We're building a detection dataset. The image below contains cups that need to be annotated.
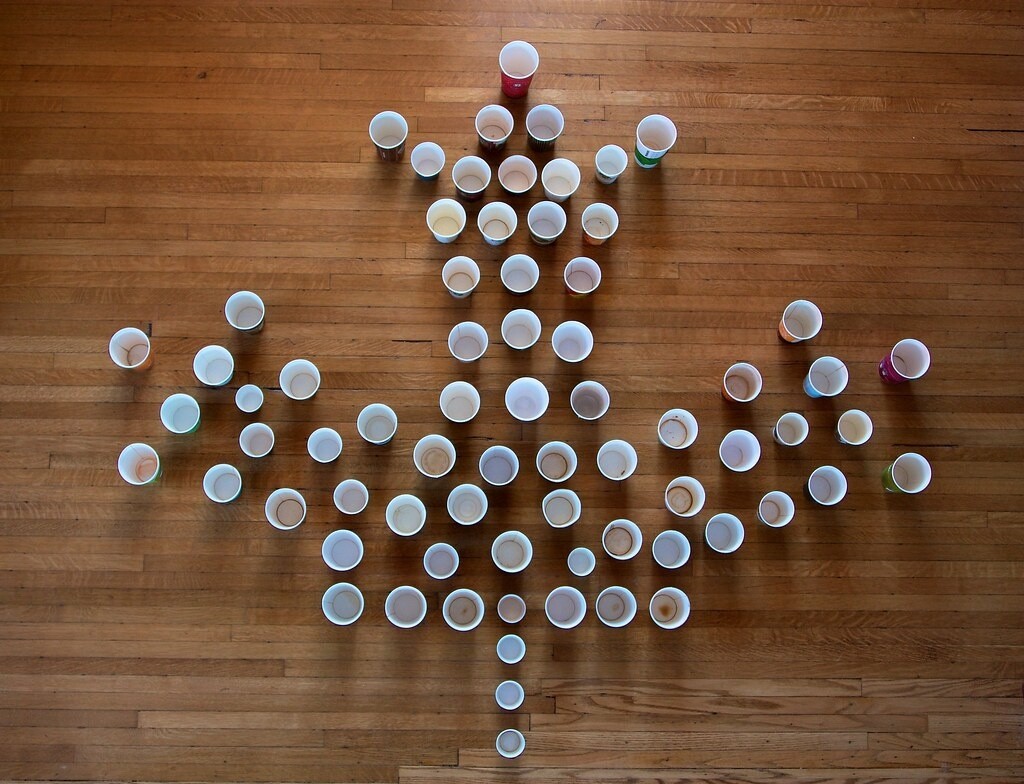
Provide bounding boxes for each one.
[109,41,932,759]
